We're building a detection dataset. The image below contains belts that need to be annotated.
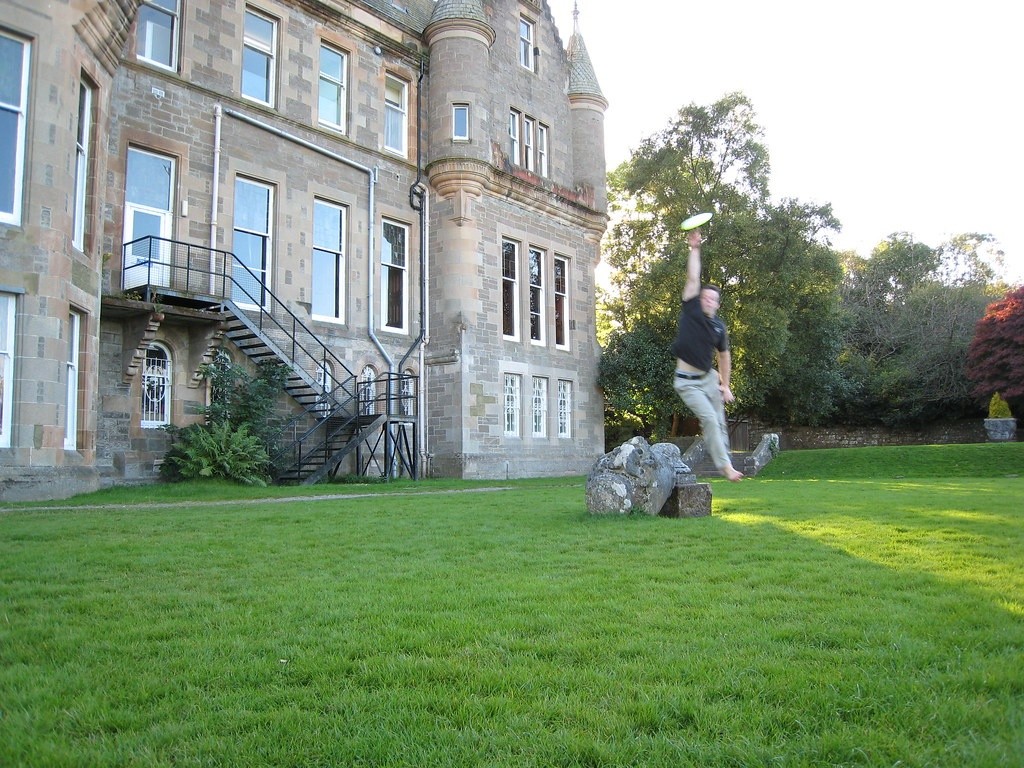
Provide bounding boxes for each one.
[674,372,708,380]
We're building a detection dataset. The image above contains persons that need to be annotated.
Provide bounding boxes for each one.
[672,228,744,483]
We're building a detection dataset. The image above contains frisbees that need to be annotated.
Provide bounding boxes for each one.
[680,213,713,231]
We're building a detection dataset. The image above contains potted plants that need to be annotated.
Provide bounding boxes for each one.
[984,392,1018,442]
